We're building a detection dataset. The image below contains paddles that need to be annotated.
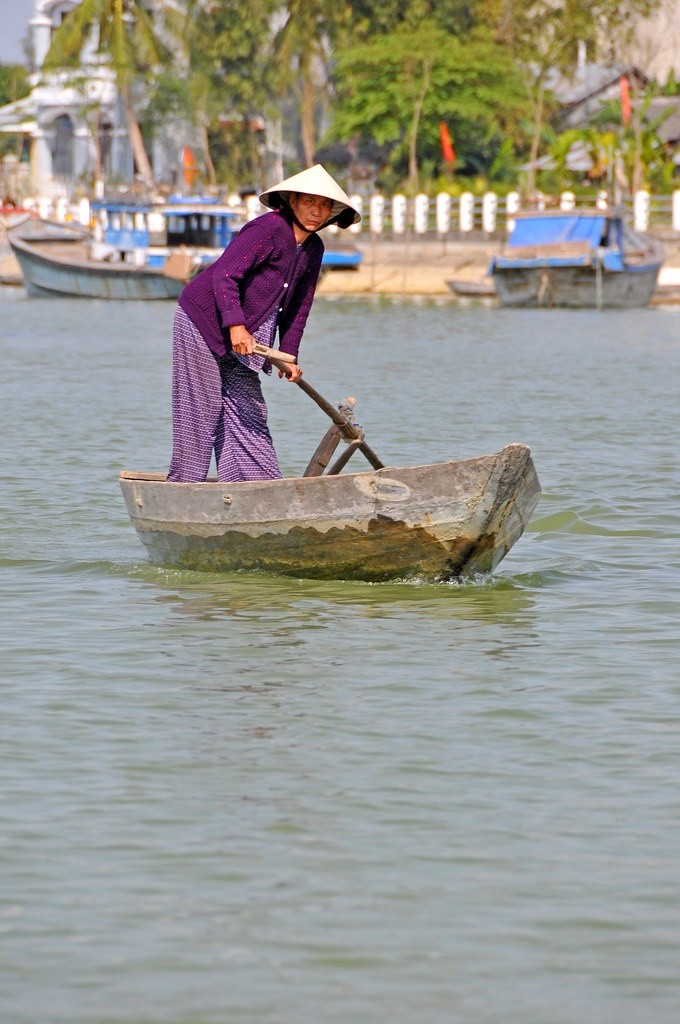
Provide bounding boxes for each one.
[251,342,384,470]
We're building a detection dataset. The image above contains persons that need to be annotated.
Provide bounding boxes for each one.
[166,163,361,482]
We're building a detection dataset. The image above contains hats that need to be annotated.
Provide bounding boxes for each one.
[257,164,362,233]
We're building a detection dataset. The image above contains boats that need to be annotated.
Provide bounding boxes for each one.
[485,210,665,306]
[118,443,542,587]
[6,204,363,299]
[446,280,494,296]
